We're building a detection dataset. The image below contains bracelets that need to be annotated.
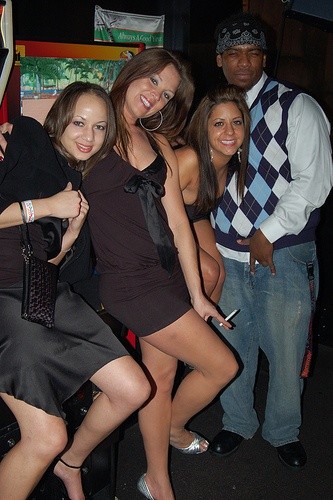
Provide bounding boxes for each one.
[18,201,26,224]
[24,200,34,223]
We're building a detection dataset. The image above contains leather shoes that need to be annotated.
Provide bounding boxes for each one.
[277,441,307,471]
[210,430,244,456]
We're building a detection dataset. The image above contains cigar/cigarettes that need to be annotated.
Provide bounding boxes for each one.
[218,309,239,326]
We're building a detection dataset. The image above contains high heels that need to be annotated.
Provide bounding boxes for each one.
[170,428,210,455]
[46,458,86,500]
[137,472,175,500]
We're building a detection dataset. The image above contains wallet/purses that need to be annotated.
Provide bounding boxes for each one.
[18,201,59,328]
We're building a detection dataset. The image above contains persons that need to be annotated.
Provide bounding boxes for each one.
[0,48,240,500]
[210,19,333,471]
[172,83,251,301]
[0,81,151,500]
